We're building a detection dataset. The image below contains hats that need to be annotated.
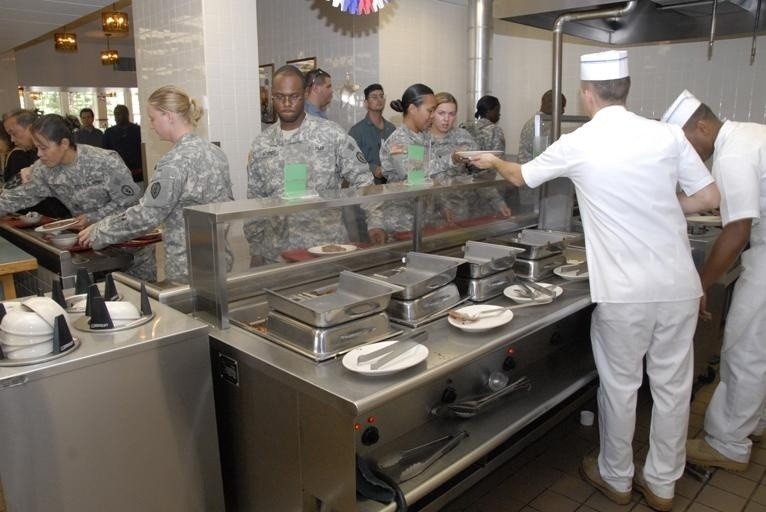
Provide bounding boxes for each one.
[660,88,702,129]
[580,50,629,81]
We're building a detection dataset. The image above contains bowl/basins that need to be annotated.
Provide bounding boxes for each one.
[50,233,79,252]
[1,296,141,364]
[19,214,43,225]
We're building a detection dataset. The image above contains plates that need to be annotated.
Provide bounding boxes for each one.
[687,215,724,227]
[142,229,162,242]
[339,264,588,378]
[307,244,357,255]
[33,217,80,234]
[454,149,504,161]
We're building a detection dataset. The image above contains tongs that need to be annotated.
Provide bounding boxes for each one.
[560,260,587,278]
[513,272,555,302]
[685,464,713,484]
[356,328,431,371]
[375,430,469,487]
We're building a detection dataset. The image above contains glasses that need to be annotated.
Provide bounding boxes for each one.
[310,68,324,87]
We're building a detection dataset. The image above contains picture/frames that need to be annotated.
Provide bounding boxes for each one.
[259,64,276,124]
[287,56,316,79]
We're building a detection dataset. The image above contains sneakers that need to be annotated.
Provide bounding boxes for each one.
[579,455,632,505]
[686,437,749,472]
[633,459,674,511]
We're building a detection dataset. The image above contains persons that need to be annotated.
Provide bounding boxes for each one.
[243,65,511,265]
[79,85,234,278]
[466,50,719,511]
[662,90,766,472]
[1,104,144,231]
[518,90,567,211]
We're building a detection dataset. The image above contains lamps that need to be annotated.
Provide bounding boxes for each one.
[102,3,129,33]
[101,34,118,65]
[55,26,77,53]
